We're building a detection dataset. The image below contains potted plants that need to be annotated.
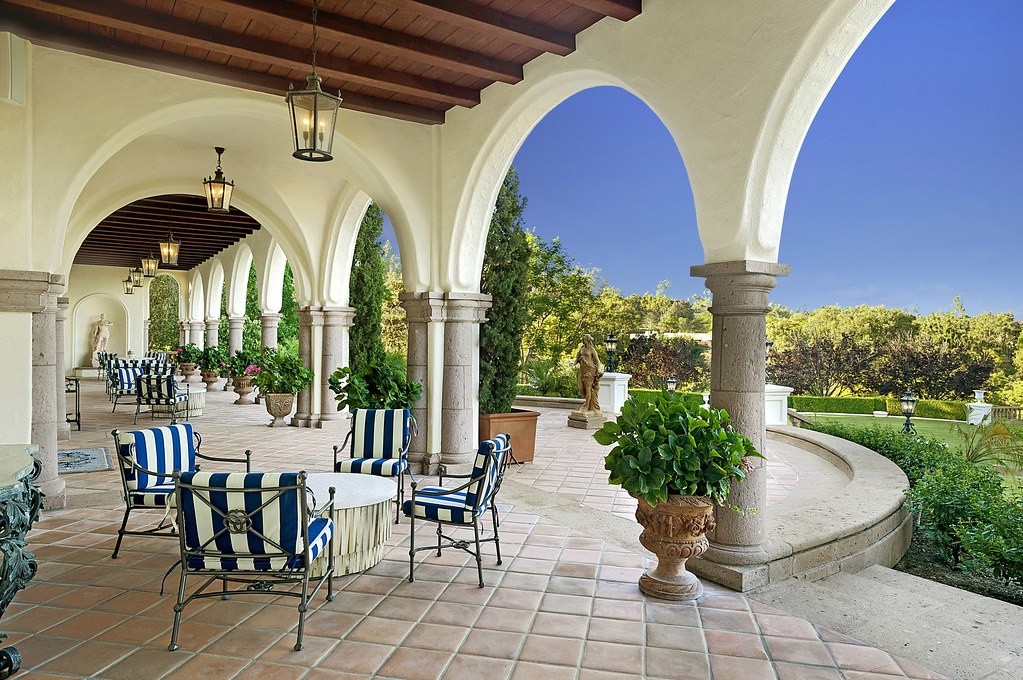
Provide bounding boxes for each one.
[198,346,226,391]
[229,350,259,405]
[173,343,203,383]
[478,167,541,467]
[591,391,767,601]
[249,355,314,428]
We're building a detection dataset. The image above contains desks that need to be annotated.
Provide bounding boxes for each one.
[303,473,399,579]
[151,383,206,418]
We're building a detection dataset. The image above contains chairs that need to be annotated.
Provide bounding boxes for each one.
[97,350,512,652]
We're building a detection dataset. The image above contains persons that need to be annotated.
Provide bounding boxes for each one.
[573,334,601,412]
[94,314,114,352]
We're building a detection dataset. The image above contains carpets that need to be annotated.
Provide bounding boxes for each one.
[58,447,116,474]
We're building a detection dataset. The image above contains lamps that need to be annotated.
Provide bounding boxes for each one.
[284,0,343,161]
[131,248,144,287]
[899,387,919,435]
[158,204,181,267]
[122,265,134,294]
[140,233,160,277]
[202,147,234,214]
